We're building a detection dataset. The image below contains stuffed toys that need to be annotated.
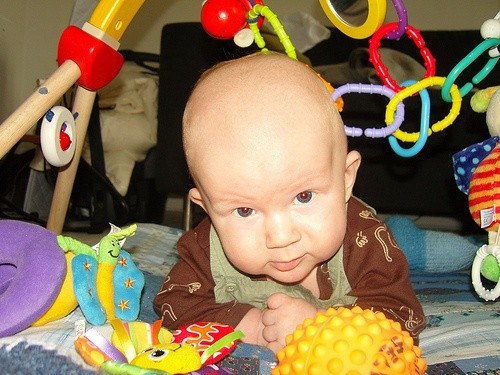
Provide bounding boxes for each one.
[454,85,500,282]
[0,219,247,375]
[56,218,145,326]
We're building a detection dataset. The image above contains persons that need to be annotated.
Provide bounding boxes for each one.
[150,50,427,345]
